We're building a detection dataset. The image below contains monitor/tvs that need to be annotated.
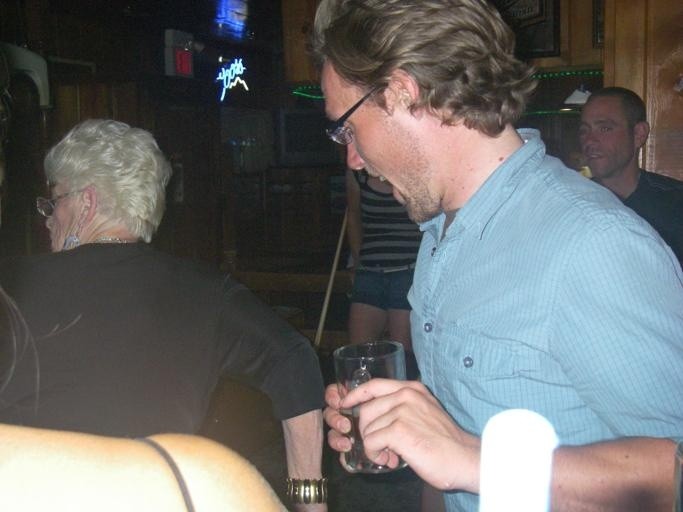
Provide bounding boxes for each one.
[212,0,249,42]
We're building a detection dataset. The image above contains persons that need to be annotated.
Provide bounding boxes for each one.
[577,86,682,270]
[0,118,329,512]
[309,0,683,512]
[0,423,289,511]
[343,164,423,378]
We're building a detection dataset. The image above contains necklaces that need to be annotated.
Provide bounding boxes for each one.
[92,236,127,243]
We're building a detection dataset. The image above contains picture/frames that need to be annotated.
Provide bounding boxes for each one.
[494,0,561,60]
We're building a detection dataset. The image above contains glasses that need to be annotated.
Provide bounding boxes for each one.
[36,193,69,217]
[324,83,387,146]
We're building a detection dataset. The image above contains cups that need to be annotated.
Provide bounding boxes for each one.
[333,340,409,474]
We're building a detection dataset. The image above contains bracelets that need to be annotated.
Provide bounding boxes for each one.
[285,478,329,504]
